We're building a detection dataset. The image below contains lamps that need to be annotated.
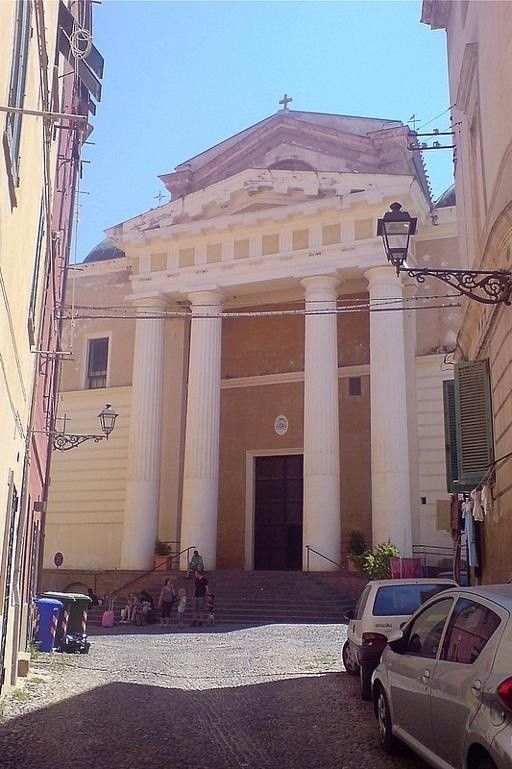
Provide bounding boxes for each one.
[375,202,512,307]
[52,403,119,453]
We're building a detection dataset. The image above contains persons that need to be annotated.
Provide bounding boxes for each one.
[190,569,209,627]
[205,592,216,627]
[88,589,96,605]
[119,592,138,624]
[127,591,153,625]
[186,551,203,579]
[175,589,187,629]
[157,578,176,628]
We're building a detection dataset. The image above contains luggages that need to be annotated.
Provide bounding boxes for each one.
[101,599,115,627]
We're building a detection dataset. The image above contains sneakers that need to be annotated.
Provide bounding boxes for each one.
[118,621,127,625]
[160,622,216,629]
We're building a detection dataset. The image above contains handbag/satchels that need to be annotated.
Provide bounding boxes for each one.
[172,594,177,602]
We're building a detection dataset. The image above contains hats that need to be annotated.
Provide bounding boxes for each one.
[206,592,213,597]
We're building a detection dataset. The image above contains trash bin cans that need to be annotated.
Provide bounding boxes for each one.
[41,590,76,648]
[63,592,92,641]
[32,597,63,653]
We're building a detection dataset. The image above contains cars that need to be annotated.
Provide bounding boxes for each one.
[342,578,460,699]
[371,584,512,768]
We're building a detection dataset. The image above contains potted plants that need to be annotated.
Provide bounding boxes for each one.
[345,530,366,573]
[154,542,173,571]
